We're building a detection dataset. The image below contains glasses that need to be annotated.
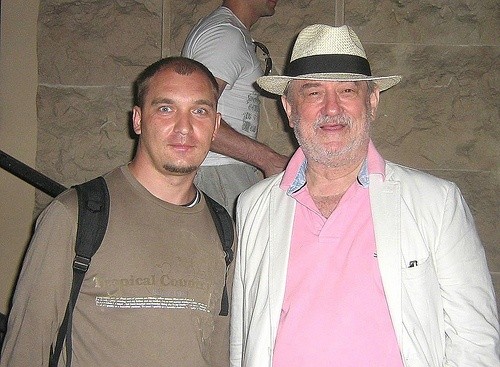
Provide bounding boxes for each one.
[253,40,273,76]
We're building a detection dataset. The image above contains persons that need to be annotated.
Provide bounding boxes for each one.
[229,23,500,367]
[0,56,239,367]
[179,0,291,222]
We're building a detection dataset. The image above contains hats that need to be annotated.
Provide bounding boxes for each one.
[256,24,403,95]
